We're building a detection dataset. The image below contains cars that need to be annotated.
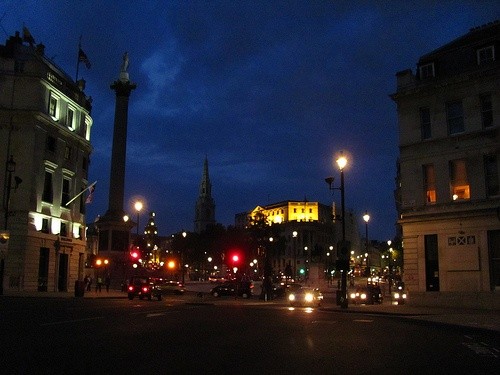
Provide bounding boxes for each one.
[289,287,324,308]
[394,281,406,305]
[127,276,186,301]
[351,284,384,305]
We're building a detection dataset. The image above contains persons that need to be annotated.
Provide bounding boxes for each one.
[105,276,112,292]
[84,274,93,291]
[95,275,103,293]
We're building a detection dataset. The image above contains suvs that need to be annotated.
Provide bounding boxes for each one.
[209,279,255,299]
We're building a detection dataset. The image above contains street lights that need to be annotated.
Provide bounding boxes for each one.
[324,150,349,309]
[133,199,143,276]
[122,215,129,273]
[361,209,371,304]
[181,231,187,285]
[387,239,393,295]
[291,229,298,291]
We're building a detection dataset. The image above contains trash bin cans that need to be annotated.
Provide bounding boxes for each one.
[336,290,342,305]
[75,280,84,297]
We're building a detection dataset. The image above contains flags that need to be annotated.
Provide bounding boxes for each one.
[79,49,92,70]
[86,183,95,204]
[22,27,35,45]
